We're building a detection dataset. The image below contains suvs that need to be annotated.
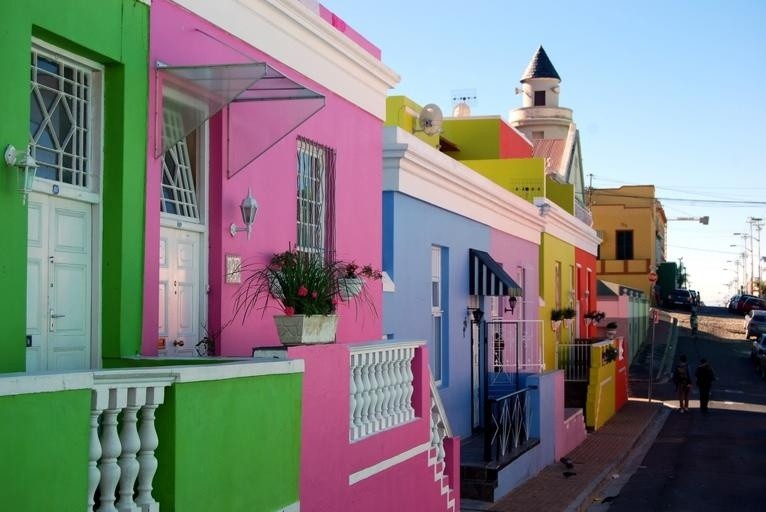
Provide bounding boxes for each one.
[744,311,766,340]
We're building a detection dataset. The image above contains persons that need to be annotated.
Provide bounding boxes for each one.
[671,355,692,414]
[695,358,716,414]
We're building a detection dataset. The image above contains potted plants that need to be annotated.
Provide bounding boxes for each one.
[592,312,605,326]
[563,308,577,328]
[217,242,379,344]
[550,308,564,330]
[584,311,596,326]
[337,261,383,301]
[606,322,617,338]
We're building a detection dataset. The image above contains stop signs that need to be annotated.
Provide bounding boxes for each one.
[648,273,657,282]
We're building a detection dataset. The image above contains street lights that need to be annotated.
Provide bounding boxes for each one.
[678,257,687,289]
[723,216,762,299]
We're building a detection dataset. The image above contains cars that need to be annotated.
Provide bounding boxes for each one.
[668,290,698,309]
[729,293,765,313]
[752,332,766,364]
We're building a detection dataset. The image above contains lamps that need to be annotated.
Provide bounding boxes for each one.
[471,308,484,328]
[505,296,517,315]
[5,144,39,204]
[229,187,258,240]
